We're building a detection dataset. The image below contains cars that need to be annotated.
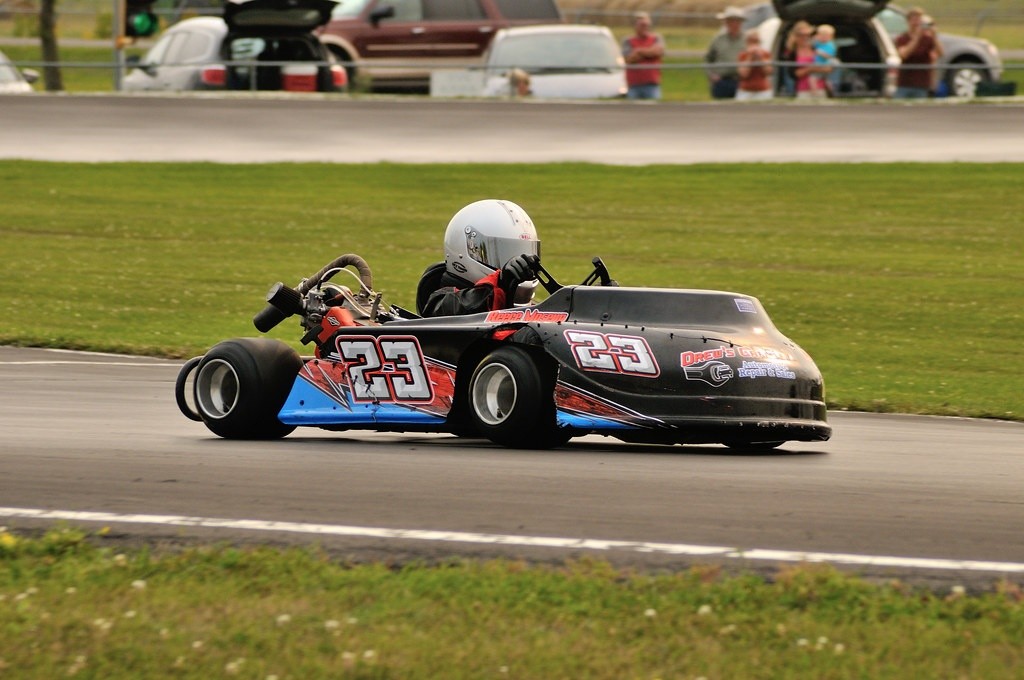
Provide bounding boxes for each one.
[742,1,1005,98]
[478,25,630,99]
[0,51,40,95]
[117,19,346,94]
[769,0,901,99]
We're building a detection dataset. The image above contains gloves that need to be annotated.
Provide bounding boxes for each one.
[497,253,540,292]
[595,279,619,286]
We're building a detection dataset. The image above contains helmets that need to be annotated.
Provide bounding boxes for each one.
[444,199,540,289]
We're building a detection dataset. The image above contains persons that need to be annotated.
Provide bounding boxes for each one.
[621,11,666,100]
[416,199,619,317]
[702,0,944,101]
[510,70,533,96]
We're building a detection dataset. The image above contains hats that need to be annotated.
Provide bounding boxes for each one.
[715,7,748,20]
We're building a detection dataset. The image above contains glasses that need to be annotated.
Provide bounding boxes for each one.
[796,32,815,38]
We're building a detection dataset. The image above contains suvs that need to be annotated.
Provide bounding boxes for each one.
[313,0,570,94]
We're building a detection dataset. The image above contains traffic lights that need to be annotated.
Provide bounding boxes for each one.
[116,0,157,46]
